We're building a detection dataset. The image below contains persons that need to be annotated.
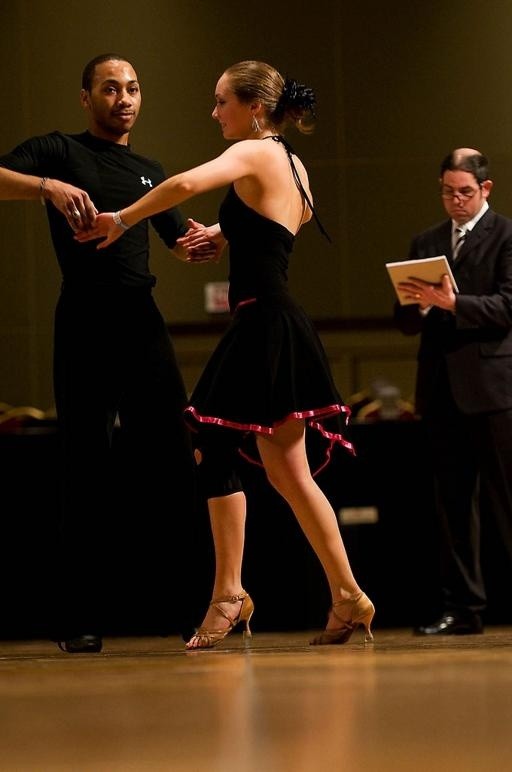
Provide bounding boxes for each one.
[0,54,217,652]
[395,148,512,636]
[74,60,377,650]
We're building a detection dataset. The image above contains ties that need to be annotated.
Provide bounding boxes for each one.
[448,229,465,259]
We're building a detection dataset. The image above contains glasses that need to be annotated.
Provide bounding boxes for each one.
[439,179,483,202]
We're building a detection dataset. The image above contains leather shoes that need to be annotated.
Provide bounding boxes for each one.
[61,631,104,653]
[411,602,485,638]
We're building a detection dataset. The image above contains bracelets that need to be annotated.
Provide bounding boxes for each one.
[112,211,130,230]
[40,177,47,201]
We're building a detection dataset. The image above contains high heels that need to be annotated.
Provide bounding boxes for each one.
[307,588,377,647]
[181,586,256,650]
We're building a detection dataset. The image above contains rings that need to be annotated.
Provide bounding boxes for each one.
[416,293,420,298]
[72,210,80,218]
